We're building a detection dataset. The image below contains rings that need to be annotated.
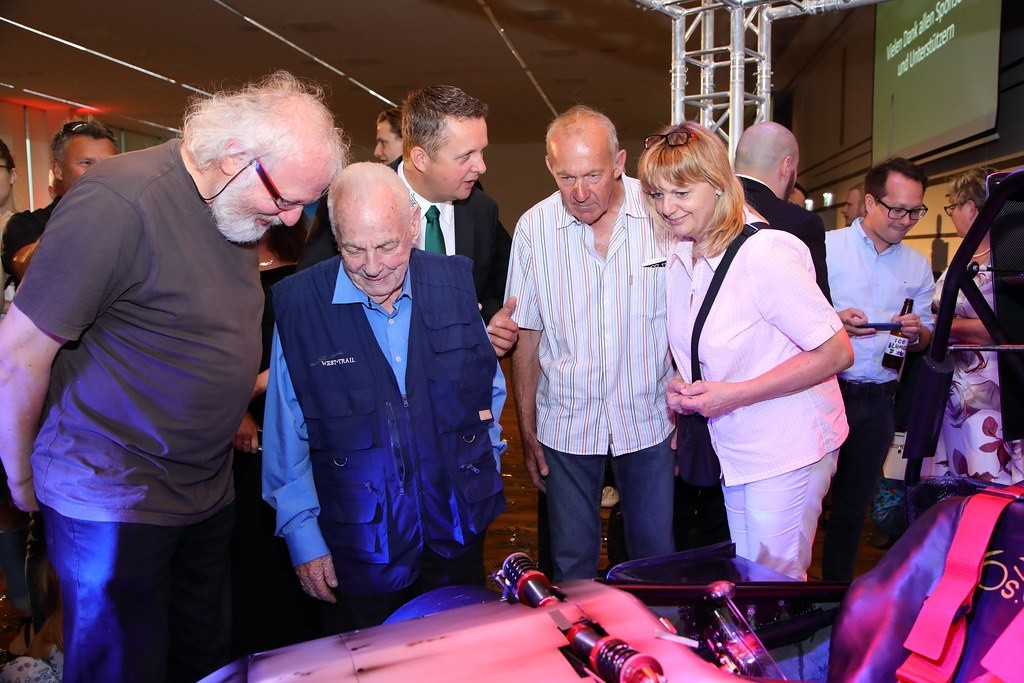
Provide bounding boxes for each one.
[242,441,250,447]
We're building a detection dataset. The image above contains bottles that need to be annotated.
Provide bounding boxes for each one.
[2,281,15,314]
[882,298,915,370]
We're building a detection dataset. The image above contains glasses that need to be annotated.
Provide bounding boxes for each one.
[253,160,328,211]
[871,192,928,220]
[945,202,963,217]
[53,121,113,159]
[643,131,700,146]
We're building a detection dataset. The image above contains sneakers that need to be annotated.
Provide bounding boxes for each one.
[600,486,619,507]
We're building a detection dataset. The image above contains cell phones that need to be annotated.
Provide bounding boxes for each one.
[855,322,902,332]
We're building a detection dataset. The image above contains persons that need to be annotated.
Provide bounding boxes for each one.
[639,111,855,580]
[825,155,935,580]
[24,548,66,682]
[232,209,332,657]
[1,68,368,682]
[502,98,679,582]
[387,83,517,589]
[1,114,122,635]
[263,162,507,638]
[932,165,1024,485]
[0,141,36,656]
[787,188,807,210]
[304,192,340,260]
[373,108,404,174]
[674,120,834,552]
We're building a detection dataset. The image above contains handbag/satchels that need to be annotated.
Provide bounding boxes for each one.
[675,409,721,486]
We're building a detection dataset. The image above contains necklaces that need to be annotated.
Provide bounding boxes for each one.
[973,247,990,257]
[258,254,279,267]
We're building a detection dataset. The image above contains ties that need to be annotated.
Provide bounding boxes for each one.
[423,206,446,256]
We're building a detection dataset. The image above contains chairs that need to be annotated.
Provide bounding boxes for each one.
[827,493,1024,683]
